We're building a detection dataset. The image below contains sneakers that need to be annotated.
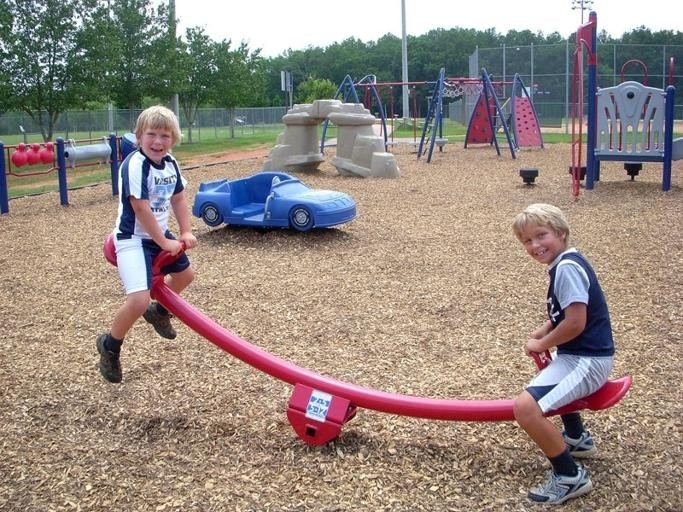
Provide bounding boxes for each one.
[142,300,177,341]
[94,333,125,383]
[557,428,600,459]
[525,464,595,506]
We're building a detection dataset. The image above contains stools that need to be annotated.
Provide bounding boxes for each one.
[104,233,187,274]
[531,350,631,414]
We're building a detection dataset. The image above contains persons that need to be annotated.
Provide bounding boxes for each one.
[93,103,199,384]
[510,200,617,508]
